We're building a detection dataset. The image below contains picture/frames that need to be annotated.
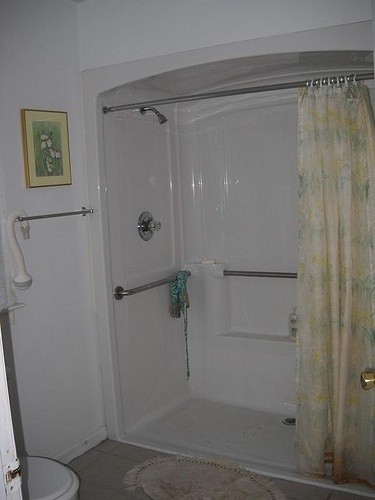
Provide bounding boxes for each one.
[21,109,72,187]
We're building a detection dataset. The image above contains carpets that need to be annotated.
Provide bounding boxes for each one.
[123,454,287,500]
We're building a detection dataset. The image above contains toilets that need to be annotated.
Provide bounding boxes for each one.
[16,455,80,500]
